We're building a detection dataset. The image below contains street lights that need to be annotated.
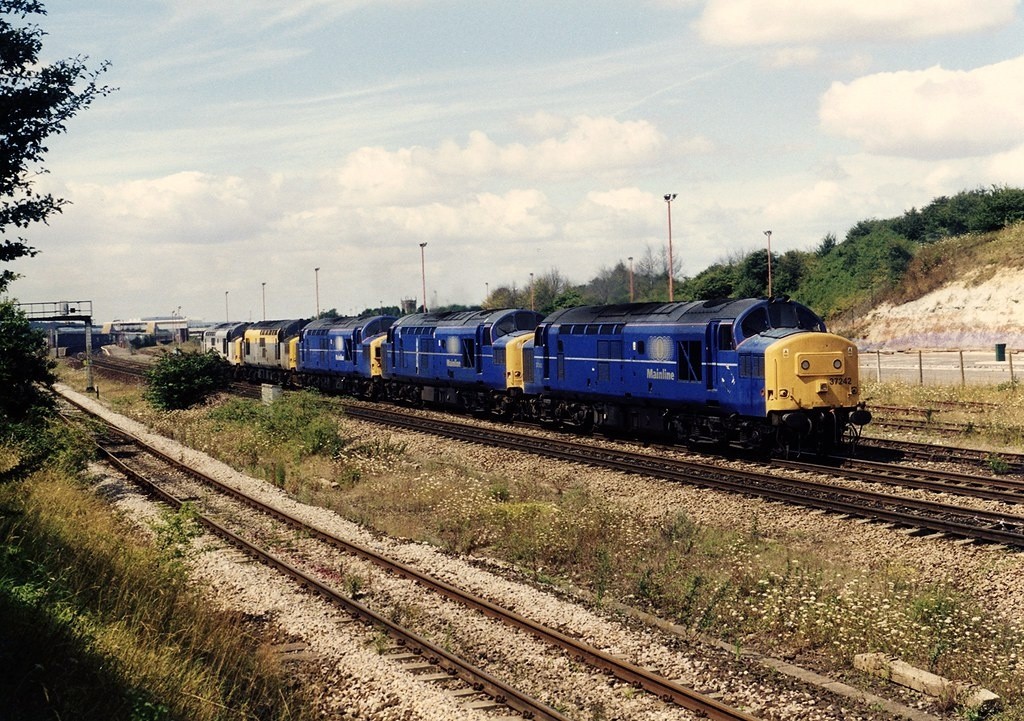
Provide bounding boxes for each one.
[663,192,679,303]
[262,282,266,321]
[627,256,634,304]
[171,306,183,322]
[419,242,427,314]
[530,272,534,311]
[315,267,320,321]
[762,228,773,296]
[225,291,228,323]
[484,282,489,299]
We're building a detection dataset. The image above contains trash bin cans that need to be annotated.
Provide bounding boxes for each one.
[995,343,1006,361]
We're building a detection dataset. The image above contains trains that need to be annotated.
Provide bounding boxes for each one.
[202,296,874,458]
